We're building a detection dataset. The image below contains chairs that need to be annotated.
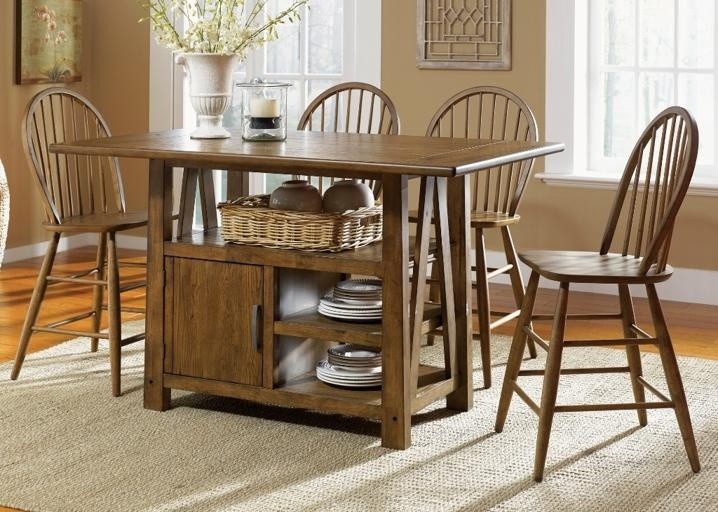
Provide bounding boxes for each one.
[495,106,702,482]
[10,86,180,396]
[408,86,538,387]
[291,81,401,200]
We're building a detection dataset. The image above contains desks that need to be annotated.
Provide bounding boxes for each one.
[47,127,565,450]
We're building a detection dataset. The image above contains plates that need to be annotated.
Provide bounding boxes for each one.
[315,345,383,389]
[318,278,383,322]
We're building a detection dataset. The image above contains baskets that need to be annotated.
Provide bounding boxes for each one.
[216,192,385,254]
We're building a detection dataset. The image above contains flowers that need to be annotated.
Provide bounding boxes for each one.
[131,1,314,63]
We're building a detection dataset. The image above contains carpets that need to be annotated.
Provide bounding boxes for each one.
[0,318,716,511]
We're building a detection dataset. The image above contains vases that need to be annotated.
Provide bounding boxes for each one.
[176,54,243,138]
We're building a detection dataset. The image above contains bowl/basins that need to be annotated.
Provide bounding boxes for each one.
[269,180,374,212]
